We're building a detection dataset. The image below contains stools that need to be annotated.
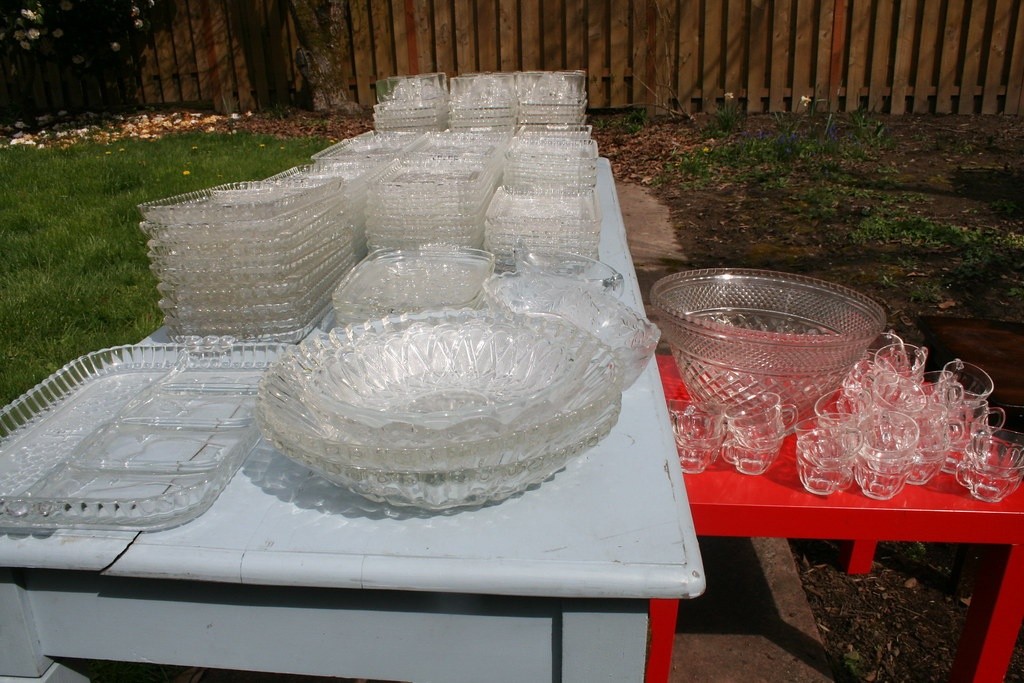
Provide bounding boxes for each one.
[913,313,1024,598]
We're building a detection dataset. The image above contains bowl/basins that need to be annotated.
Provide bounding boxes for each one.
[649,266,887,425]
[256,311,626,512]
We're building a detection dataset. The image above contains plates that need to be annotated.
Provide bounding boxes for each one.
[141,65,661,391]
[1,337,298,535]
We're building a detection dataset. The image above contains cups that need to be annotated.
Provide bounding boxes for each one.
[683,330,1024,503]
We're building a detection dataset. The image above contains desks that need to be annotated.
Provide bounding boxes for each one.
[0,155,707,683]
[643,356,1024,683]
